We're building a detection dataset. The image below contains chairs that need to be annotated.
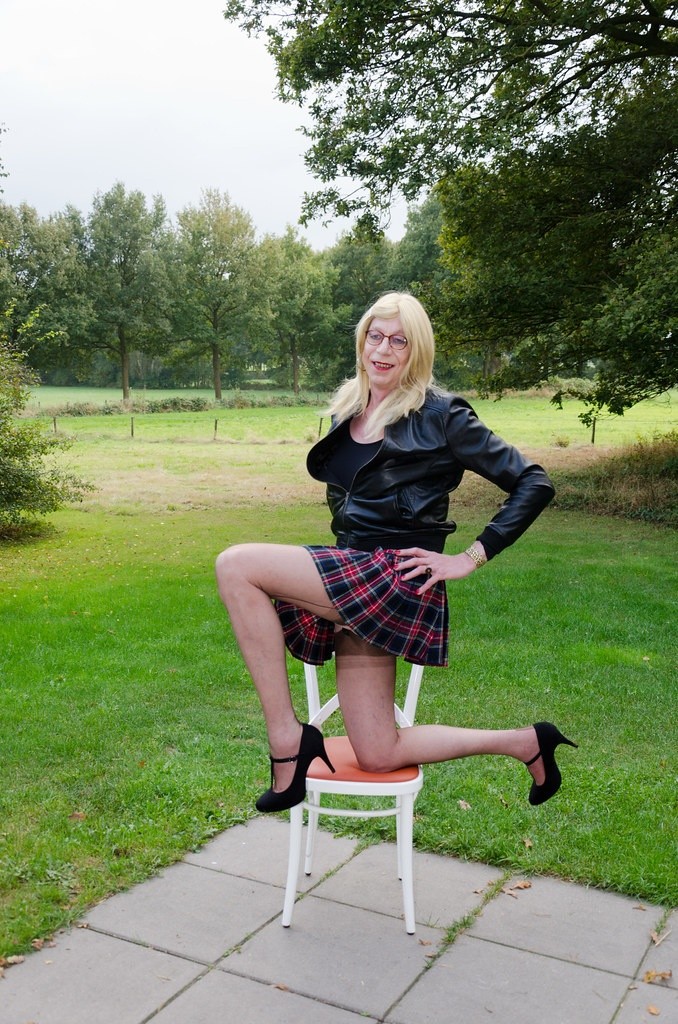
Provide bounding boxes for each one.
[281,653,424,937]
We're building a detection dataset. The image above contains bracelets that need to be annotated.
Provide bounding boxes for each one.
[465,547,485,568]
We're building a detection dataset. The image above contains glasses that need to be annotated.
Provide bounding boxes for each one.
[361,327,412,352]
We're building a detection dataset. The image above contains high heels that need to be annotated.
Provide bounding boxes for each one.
[524,722,579,805]
[255,723,336,814]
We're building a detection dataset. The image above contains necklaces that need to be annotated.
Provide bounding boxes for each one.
[365,410,370,420]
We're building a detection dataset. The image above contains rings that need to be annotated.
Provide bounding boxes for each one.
[425,565,432,574]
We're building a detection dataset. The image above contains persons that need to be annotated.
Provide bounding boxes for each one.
[216,294,579,812]
